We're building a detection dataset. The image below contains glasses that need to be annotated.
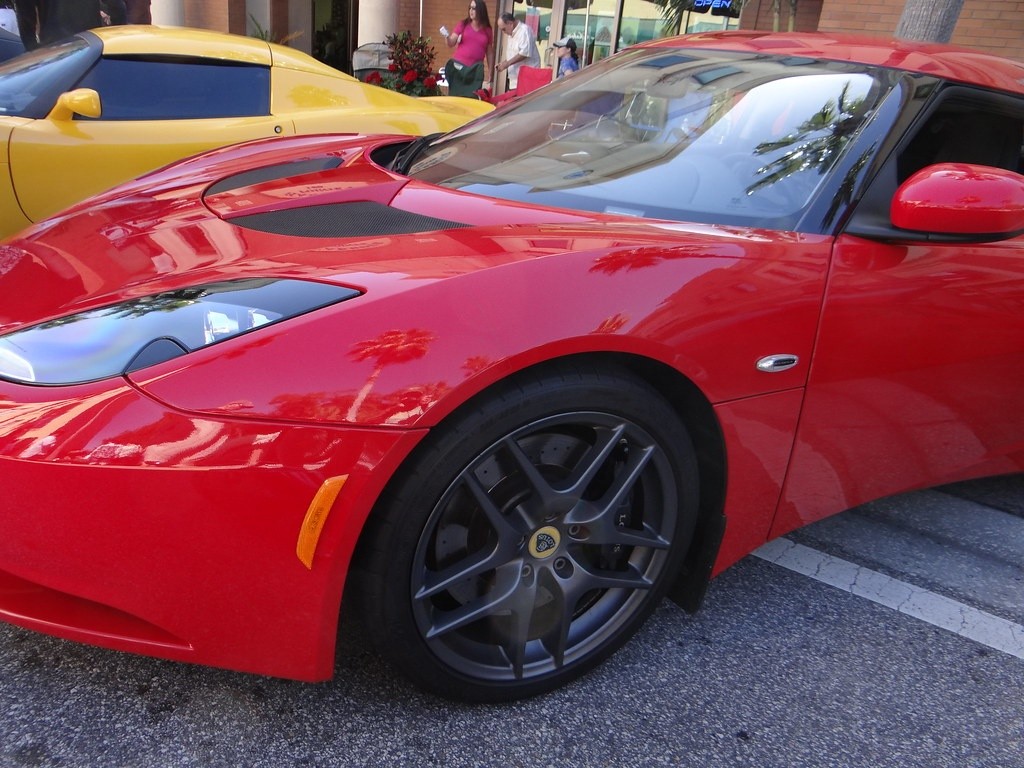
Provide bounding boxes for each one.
[468,6,476,10]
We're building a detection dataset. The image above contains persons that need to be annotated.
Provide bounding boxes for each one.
[495,12,541,90]
[319,31,336,65]
[15,0,129,51]
[440,0,493,98]
[587,26,640,66]
[553,37,579,77]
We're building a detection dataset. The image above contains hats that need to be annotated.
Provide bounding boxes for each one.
[553,37,577,50]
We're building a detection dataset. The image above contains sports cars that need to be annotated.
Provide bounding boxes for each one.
[0,25,503,242]
[0,31,1024,709]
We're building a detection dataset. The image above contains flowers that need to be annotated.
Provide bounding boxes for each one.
[362,28,445,97]
